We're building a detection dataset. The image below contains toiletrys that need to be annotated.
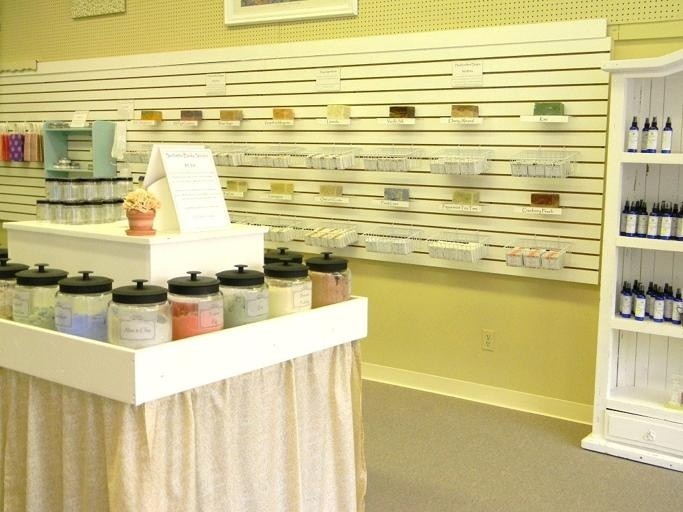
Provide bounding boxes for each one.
[617,115,683,325]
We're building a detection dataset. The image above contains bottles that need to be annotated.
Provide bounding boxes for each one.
[620,199,683,241]
[107,279,173,348]
[12,263,70,331]
[262,258,313,318]
[265,246,304,265]
[54,270,115,342]
[0,258,30,319]
[626,115,673,153]
[619,280,682,326]
[167,271,225,340]
[217,265,270,328]
[305,250,352,309]
[36,175,135,226]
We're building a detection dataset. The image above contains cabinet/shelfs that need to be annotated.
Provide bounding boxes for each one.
[580,46,682,473]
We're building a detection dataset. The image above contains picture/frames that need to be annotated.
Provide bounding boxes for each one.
[222,0,357,26]
